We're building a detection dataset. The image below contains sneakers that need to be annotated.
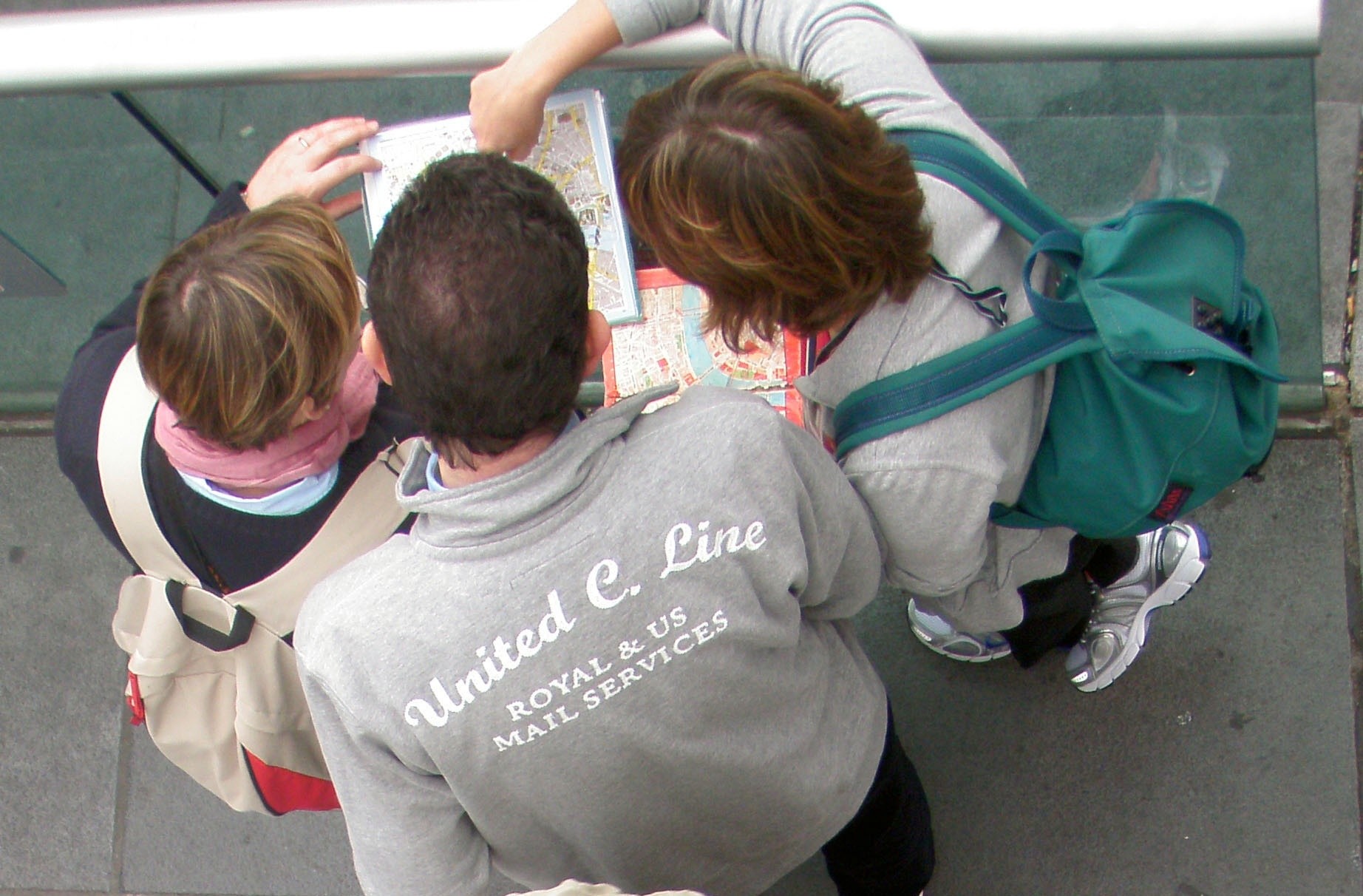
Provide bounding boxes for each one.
[906,597,1012,662]
[1065,521,1210,690]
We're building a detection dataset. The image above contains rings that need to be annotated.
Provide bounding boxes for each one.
[299,138,310,149]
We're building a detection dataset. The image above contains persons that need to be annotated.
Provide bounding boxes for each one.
[292,153,932,896]
[55,115,429,647]
[467,0,1209,692]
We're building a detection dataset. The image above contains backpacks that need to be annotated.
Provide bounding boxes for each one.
[831,126,1287,539]
[97,345,431,817]
